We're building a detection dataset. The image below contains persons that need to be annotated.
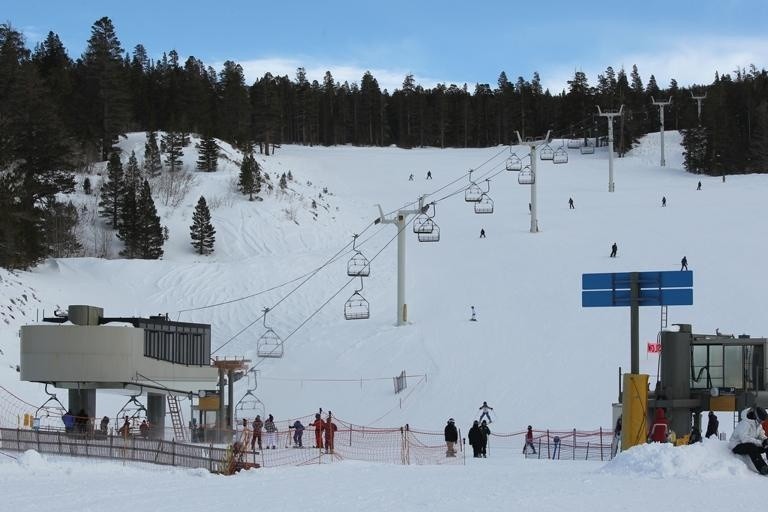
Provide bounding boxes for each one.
[409,175,414,181]
[480,229,486,238]
[468,421,482,457]
[289,421,305,448]
[523,426,536,454]
[309,414,325,448]
[529,203,531,211]
[648,408,671,443]
[445,418,457,457]
[662,197,666,207]
[100,417,109,432]
[251,415,263,450]
[705,412,719,439]
[681,256,688,271]
[728,407,768,475]
[321,418,337,454]
[610,243,617,257]
[478,420,490,458]
[479,402,493,425]
[264,414,276,449]
[62,409,75,433]
[568,198,574,209]
[118,425,130,437]
[697,181,701,190]
[75,410,88,433]
[140,420,149,437]
[427,171,432,180]
[470,306,476,321]
